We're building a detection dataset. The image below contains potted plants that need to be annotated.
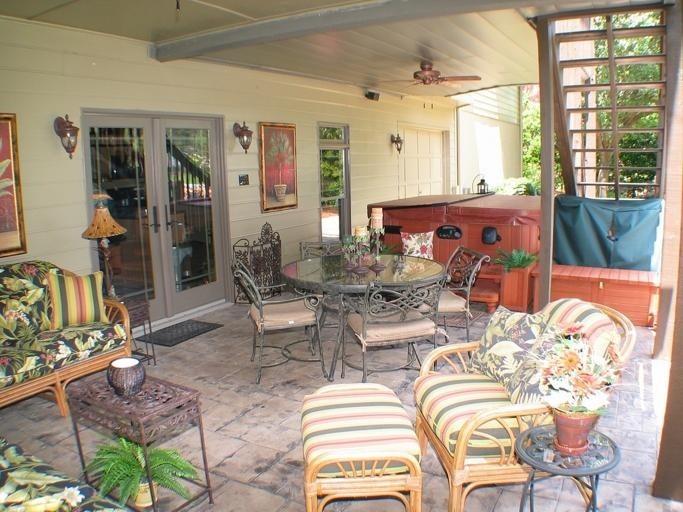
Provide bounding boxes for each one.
[78,435,207,512]
[492,244,539,312]
[263,131,294,202]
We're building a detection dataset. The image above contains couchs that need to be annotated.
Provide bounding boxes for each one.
[0,258,134,418]
[401,295,640,511]
[0,431,141,512]
[298,381,424,511]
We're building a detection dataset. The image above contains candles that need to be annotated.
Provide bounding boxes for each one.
[354,225,367,241]
[368,206,386,232]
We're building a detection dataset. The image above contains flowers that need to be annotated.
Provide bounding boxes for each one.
[515,315,638,415]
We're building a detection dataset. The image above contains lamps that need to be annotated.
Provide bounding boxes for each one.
[470,172,489,196]
[80,199,128,310]
[388,132,404,155]
[50,114,80,161]
[232,121,253,155]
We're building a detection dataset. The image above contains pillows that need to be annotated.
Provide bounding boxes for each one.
[512,335,572,411]
[398,230,437,260]
[40,270,112,331]
[467,306,549,396]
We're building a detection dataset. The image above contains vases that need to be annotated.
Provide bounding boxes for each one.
[550,404,602,458]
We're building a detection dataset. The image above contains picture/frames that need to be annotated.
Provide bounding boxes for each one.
[0,111,27,259]
[253,118,302,213]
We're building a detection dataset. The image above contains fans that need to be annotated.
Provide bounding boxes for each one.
[375,56,482,94]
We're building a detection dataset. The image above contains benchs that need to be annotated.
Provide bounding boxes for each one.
[530,257,659,327]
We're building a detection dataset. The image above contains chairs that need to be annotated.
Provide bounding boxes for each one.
[288,237,363,356]
[320,271,449,385]
[227,260,329,389]
[401,243,490,365]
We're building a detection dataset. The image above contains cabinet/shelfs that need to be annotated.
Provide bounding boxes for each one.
[366,194,542,310]
[99,297,156,371]
[60,370,214,512]
[232,242,282,304]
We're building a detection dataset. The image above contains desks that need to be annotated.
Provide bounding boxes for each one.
[510,421,621,511]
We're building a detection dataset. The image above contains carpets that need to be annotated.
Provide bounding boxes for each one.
[133,318,224,348]
